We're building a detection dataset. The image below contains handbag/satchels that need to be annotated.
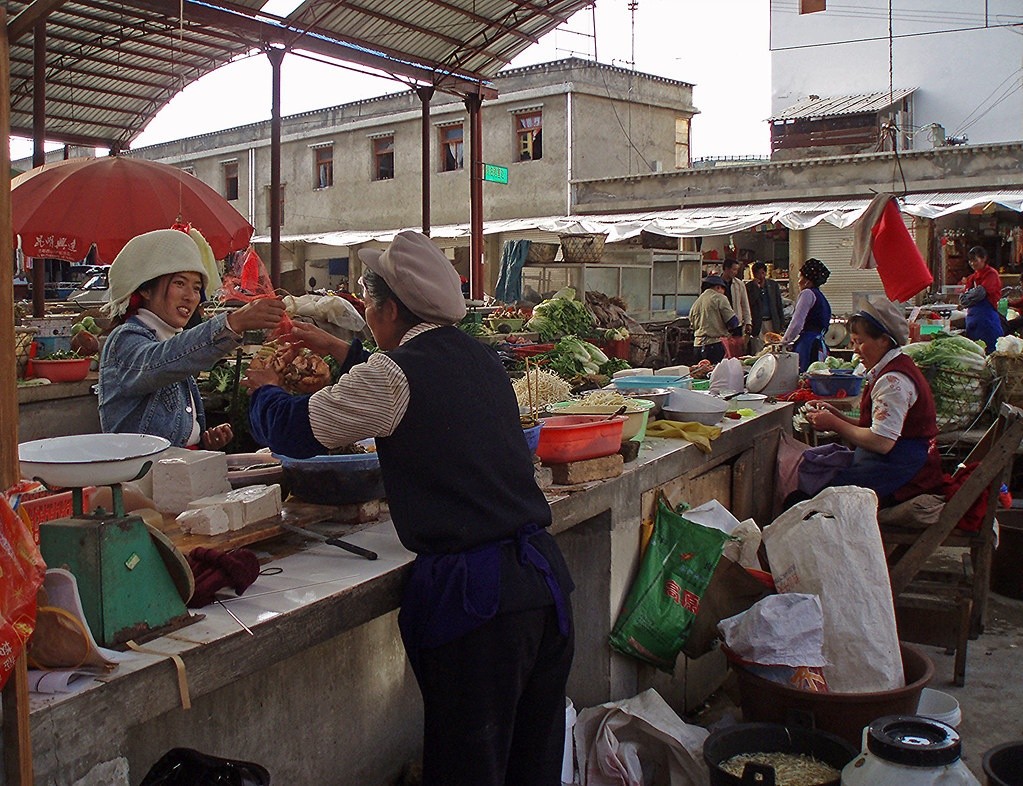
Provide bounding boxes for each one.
[709,357,745,396]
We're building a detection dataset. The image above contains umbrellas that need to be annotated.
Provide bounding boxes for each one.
[11,153,255,264]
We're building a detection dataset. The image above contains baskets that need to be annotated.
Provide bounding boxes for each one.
[557,230,610,262]
[527,242,561,262]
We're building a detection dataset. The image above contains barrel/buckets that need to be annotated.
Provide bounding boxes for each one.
[981,741,1023,786]
[841,714,982,786]
[704,718,862,786]
[731,632,934,745]
[916,688,961,730]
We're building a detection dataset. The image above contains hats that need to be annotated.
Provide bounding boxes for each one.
[358,230,467,325]
[799,258,830,285]
[859,296,911,347]
[700,275,727,289]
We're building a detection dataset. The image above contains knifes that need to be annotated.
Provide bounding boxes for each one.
[281,523,378,560]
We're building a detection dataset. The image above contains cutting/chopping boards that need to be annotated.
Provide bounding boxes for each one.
[154,501,338,560]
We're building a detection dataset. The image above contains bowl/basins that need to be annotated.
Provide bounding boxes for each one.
[30,358,93,382]
[489,318,524,332]
[537,375,768,463]
[920,325,945,335]
[275,451,382,503]
[226,452,287,497]
[805,369,864,397]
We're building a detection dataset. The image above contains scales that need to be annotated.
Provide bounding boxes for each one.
[933,305,958,332]
[746,338,800,399]
[823,312,853,349]
[19,433,194,645]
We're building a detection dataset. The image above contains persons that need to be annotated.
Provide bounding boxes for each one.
[746,263,785,338]
[522,285,541,302]
[783,296,937,512]
[957,246,1003,355]
[1007,273,1023,336]
[782,258,831,371]
[239,231,577,785]
[99,230,285,451]
[719,260,753,358]
[689,276,738,364]
[954,277,967,294]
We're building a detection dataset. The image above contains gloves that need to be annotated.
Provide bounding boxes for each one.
[645,419,723,453]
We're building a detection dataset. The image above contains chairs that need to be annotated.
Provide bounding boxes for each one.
[876,403,1023,637]
